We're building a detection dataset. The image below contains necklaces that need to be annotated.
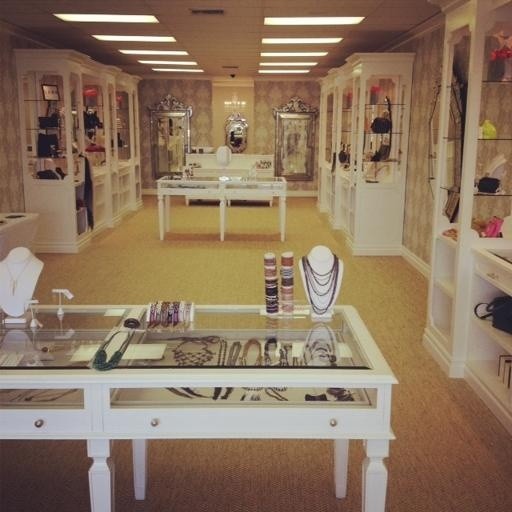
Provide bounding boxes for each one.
[303,321,342,368]
[3,258,38,300]
[0,389,80,403]
[298,253,344,314]
[91,329,354,401]
[146,296,192,329]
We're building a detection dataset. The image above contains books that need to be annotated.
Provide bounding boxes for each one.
[496,352,511,389]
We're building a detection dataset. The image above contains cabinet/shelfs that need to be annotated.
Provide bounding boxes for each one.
[283,117,306,175]
[316,68,337,219]
[184,153,274,208]
[420,0,512,437]
[113,65,144,230]
[329,53,414,258]
[0,302,398,512]
[158,173,289,241]
[155,113,183,170]
[11,47,112,255]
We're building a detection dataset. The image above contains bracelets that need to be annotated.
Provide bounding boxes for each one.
[261,250,296,314]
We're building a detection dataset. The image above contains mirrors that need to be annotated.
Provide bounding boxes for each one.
[150,111,191,177]
[225,112,249,153]
[276,111,314,182]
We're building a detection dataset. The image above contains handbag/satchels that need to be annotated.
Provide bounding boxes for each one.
[38,133,59,157]
[474,296,511,333]
[371,117,390,133]
[39,113,58,129]
[83,111,100,129]
[379,144,390,160]
